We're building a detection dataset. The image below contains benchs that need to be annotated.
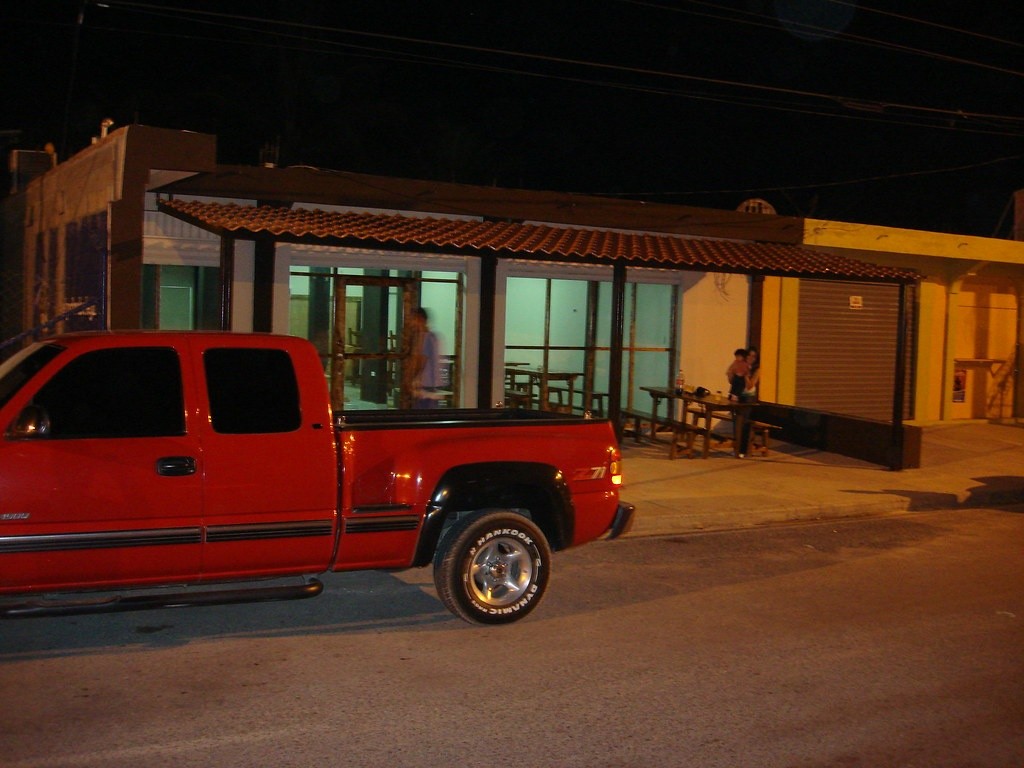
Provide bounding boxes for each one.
[348,328,783,460]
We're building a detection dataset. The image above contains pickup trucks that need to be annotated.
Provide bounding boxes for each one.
[0,330,637,628]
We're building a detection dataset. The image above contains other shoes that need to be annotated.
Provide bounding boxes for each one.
[731,394,739,402]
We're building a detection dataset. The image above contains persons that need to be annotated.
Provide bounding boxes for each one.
[402,308,441,410]
[726,346,761,457]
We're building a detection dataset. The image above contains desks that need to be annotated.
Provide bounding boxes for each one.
[639,386,776,459]
[437,361,531,391]
[504,367,583,415]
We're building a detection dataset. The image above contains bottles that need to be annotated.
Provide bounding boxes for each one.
[715,391,722,402]
[676,370,684,396]
